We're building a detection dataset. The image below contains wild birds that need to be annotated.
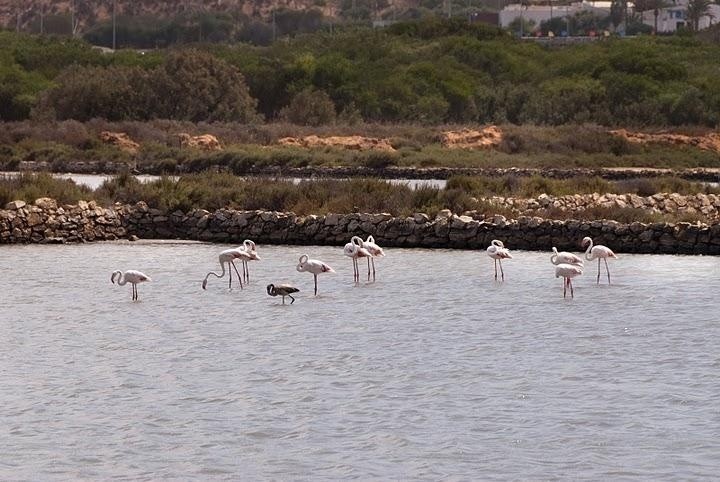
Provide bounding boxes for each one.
[344,236,386,283]
[202,239,262,290]
[111,270,151,301]
[267,283,300,305]
[487,240,513,283]
[550,236,617,300]
[296,254,335,296]
